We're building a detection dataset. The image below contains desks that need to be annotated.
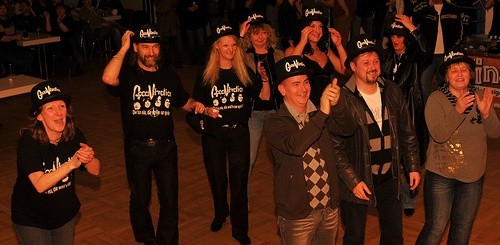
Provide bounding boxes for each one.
[0,74,47,120]
[17,33,61,80]
[102,15,122,53]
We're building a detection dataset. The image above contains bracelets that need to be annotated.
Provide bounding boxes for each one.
[58,22,62,24]
[113,55,123,64]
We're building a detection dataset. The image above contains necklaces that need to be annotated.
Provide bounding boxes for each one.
[50,136,63,143]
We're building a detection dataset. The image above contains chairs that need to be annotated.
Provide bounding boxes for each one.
[71,19,114,64]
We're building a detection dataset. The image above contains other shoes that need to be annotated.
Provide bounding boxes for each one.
[404,208,415,217]
[211,217,226,232]
[232,233,251,245]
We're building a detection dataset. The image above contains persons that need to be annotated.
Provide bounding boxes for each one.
[238,13,285,178]
[379,15,433,217]
[282,19,348,103]
[0,0,117,79]
[153,0,500,81]
[11,82,100,245]
[414,52,500,245]
[263,56,373,245]
[188,23,271,245]
[328,51,421,245]
[100,25,206,245]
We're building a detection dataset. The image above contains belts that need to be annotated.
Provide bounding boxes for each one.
[220,124,238,130]
[130,138,166,148]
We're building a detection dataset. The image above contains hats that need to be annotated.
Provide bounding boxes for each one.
[30,81,71,110]
[244,12,272,26]
[301,6,328,25]
[387,21,409,36]
[208,23,239,47]
[438,45,476,76]
[344,35,379,71]
[272,56,309,82]
[130,24,163,43]
